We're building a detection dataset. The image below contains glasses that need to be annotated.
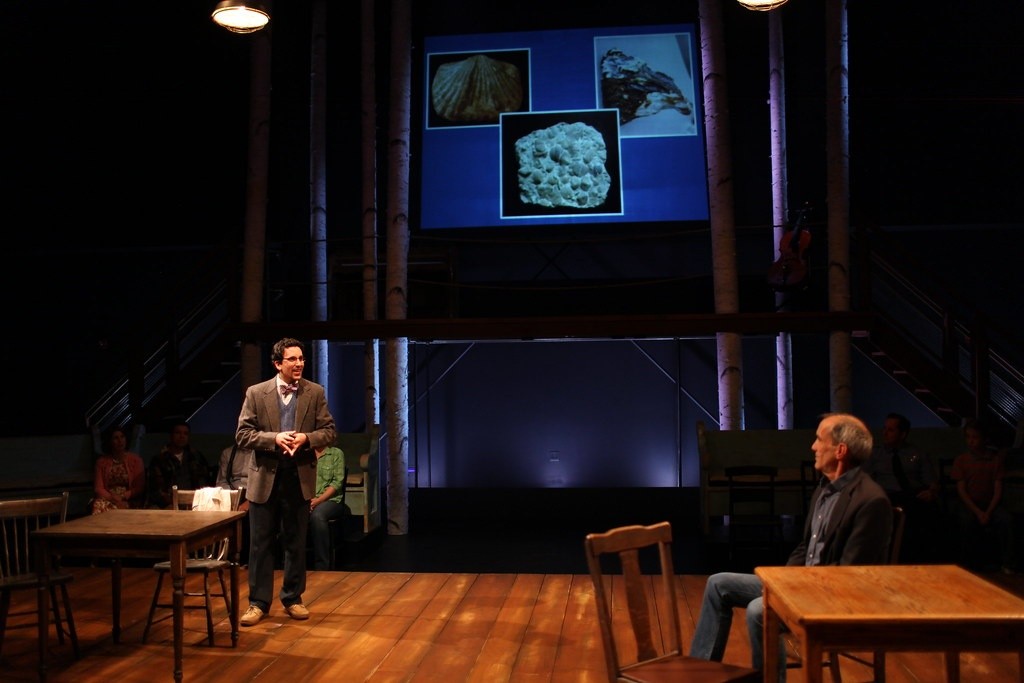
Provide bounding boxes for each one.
[284,356,304,362]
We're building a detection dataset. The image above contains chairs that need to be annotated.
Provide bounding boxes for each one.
[0,491,80,662]
[723,465,784,565]
[141,485,246,646]
[785,503,904,683]
[585,521,762,683]
[800,458,821,525]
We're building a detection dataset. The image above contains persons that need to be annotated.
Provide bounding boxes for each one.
[691,410,892,683]
[91,426,146,515]
[877,408,940,567]
[305,444,346,571]
[234,335,337,628]
[146,421,209,508]
[216,443,252,559]
[948,418,1004,574]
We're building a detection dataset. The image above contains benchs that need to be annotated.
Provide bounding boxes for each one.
[127,430,384,534]
[695,426,970,536]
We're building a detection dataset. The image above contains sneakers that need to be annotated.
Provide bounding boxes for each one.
[285,604,308,620]
[240,604,270,625]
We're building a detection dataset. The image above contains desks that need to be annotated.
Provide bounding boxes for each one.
[753,563,1024,683]
[30,508,251,683]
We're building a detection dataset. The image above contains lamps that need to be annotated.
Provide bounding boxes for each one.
[737,0,789,12]
[210,0,272,34]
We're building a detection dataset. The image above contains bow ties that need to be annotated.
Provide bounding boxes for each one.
[280,384,299,394]
